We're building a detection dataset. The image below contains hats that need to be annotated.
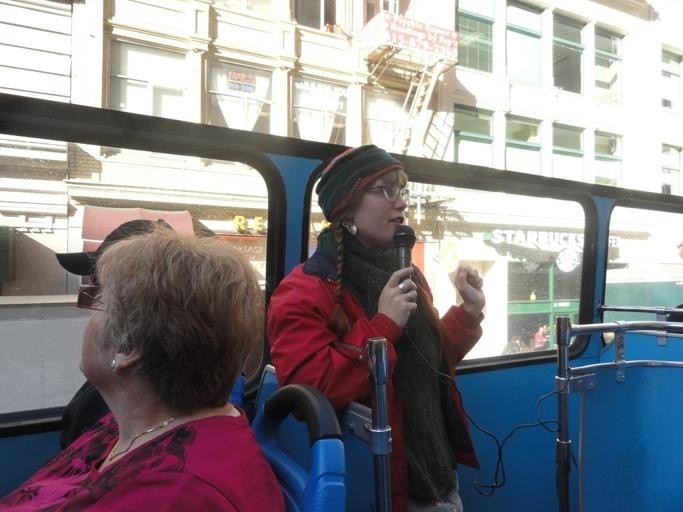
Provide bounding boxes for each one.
[315,144,405,222]
[56,219,176,276]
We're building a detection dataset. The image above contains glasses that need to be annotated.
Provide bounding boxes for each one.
[365,183,412,206]
[75,286,106,312]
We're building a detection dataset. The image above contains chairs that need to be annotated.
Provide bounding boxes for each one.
[251,383,349,512]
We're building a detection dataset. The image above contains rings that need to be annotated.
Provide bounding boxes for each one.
[408,291,415,301]
[398,282,408,292]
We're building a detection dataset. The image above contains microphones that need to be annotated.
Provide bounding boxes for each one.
[392,224,416,329]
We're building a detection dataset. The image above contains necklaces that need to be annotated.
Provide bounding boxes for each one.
[108,414,178,460]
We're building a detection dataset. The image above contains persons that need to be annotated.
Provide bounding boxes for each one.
[60,219,174,449]
[503,322,551,354]
[265,146,485,512]
[0,230,287,512]
